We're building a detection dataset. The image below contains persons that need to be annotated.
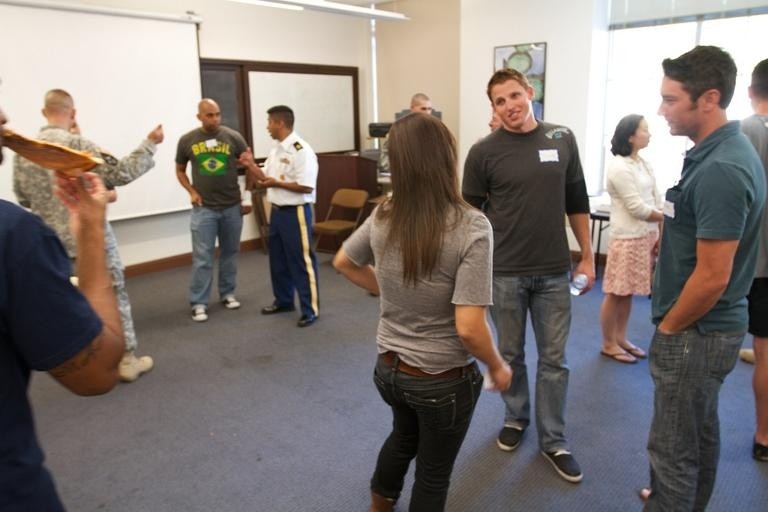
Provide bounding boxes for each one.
[462,69,596,483]
[238,105,320,327]
[378,93,432,173]
[175,98,255,322]
[0,103,125,512]
[490,111,502,134]
[12,89,163,383]
[740,60,768,462]
[601,115,664,364]
[331,110,512,512]
[641,45,768,512]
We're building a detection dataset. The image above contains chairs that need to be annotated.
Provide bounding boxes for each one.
[310,189,370,274]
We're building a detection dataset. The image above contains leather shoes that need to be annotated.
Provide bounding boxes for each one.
[296,313,318,328]
[261,301,295,314]
[738,347,756,363]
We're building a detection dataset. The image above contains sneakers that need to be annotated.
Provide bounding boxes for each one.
[119,352,154,383]
[191,303,209,322]
[224,294,241,309]
[751,434,767,461]
[495,422,524,451]
[539,446,584,483]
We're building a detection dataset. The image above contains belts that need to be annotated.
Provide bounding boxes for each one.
[377,357,476,378]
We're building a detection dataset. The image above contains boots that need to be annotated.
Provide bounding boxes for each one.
[368,491,396,512]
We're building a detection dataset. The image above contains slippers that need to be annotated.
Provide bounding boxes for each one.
[599,347,639,364]
[627,343,647,359]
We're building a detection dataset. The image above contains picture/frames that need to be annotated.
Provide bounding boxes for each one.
[493,42,546,122]
